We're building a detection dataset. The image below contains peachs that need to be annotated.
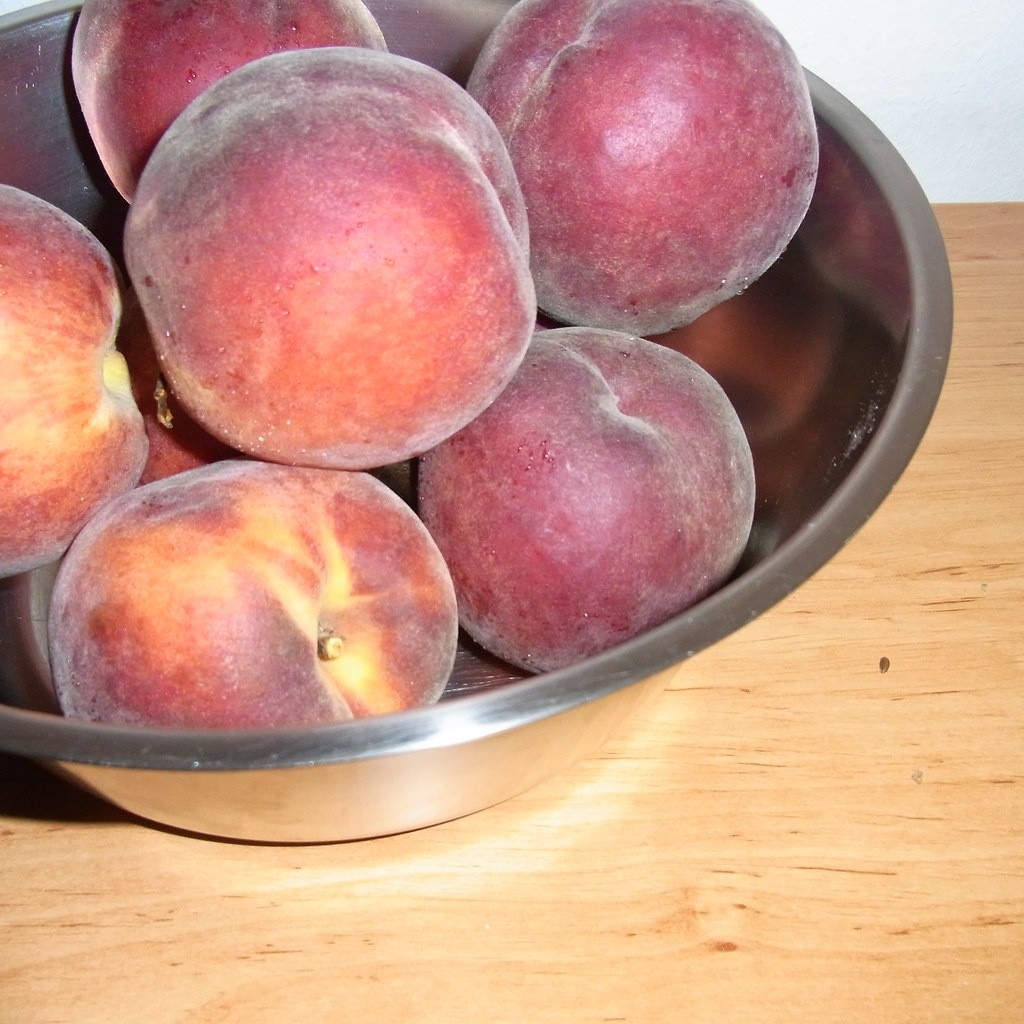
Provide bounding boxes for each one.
[0,0,822,729]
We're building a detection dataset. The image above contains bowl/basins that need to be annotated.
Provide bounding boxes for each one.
[0,0,955,845]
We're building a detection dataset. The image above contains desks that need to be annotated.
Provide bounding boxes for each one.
[0,202,1024,1024]
